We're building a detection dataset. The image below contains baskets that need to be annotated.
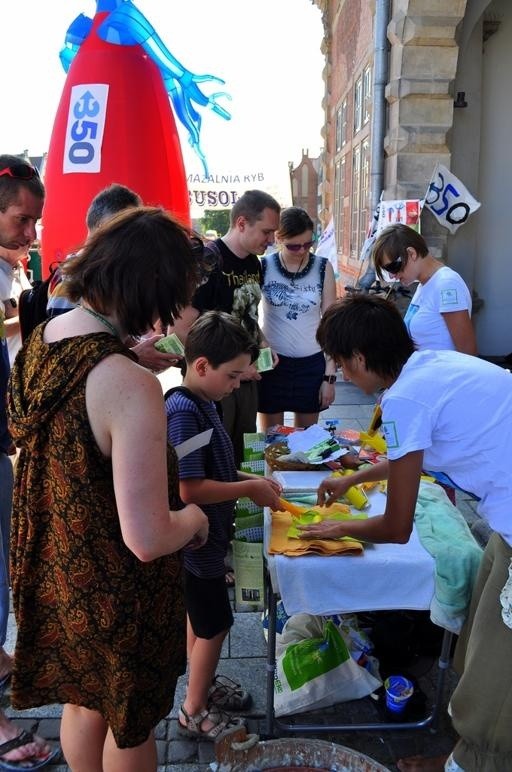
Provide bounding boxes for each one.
[264,443,321,471]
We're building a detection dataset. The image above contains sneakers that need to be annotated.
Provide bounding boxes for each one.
[396,753,448,772]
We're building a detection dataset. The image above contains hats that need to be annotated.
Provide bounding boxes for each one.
[203,230,220,241]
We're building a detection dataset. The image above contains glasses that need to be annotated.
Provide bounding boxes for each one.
[285,240,315,251]
[0,164,40,179]
[385,257,402,274]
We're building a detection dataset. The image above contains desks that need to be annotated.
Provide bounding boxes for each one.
[263,442,484,740]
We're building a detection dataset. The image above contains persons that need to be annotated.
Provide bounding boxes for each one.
[0,155,222,772]
[159,310,285,739]
[373,223,478,507]
[167,189,337,471]
[295,294,512,771]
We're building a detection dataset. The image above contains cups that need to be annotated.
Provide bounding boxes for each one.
[383,675,414,714]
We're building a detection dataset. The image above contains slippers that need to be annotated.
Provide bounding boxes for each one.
[0,671,13,696]
[0,730,61,772]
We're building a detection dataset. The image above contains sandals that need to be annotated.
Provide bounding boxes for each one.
[224,566,235,587]
[176,705,246,738]
[185,681,249,709]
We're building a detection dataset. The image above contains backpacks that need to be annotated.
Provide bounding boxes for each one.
[19,268,75,345]
